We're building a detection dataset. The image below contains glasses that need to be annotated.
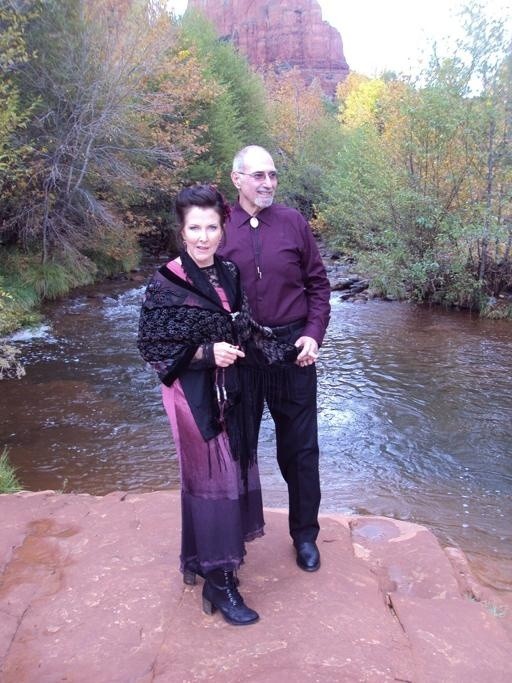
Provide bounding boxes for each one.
[237,171,277,181]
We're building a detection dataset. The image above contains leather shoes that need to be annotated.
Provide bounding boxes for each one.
[294,532,321,572]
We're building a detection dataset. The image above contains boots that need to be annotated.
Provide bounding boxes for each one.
[183,557,259,625]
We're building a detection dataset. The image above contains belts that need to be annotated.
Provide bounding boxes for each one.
[270,318,305,337]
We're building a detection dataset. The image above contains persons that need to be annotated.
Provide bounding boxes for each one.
[134,182,326,625]
[211,145,331,572]
